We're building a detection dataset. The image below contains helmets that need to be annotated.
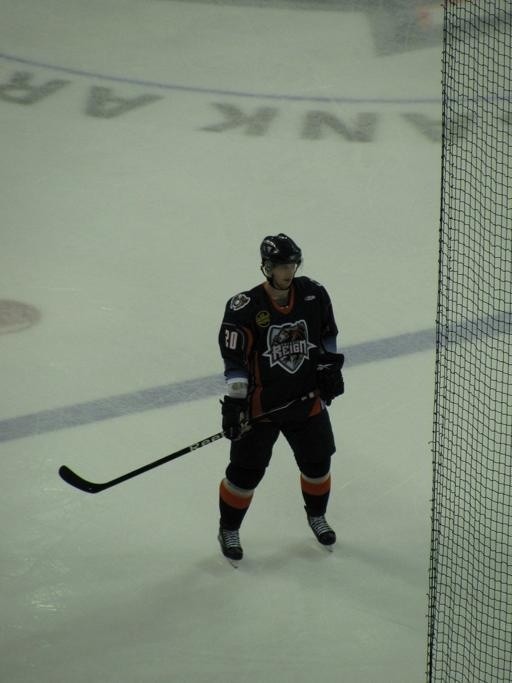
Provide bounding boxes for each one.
[260,232,302,269]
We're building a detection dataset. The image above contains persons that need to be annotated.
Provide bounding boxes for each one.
[214,230,346,562]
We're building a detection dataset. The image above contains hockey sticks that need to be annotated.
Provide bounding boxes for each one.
[59,388,317,496]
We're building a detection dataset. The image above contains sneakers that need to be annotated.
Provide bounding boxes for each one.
[304,506,337,546]
[218,522,243,560]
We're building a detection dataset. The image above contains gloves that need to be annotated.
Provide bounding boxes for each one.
[315,352,344,406]
[221,394,250,441]
